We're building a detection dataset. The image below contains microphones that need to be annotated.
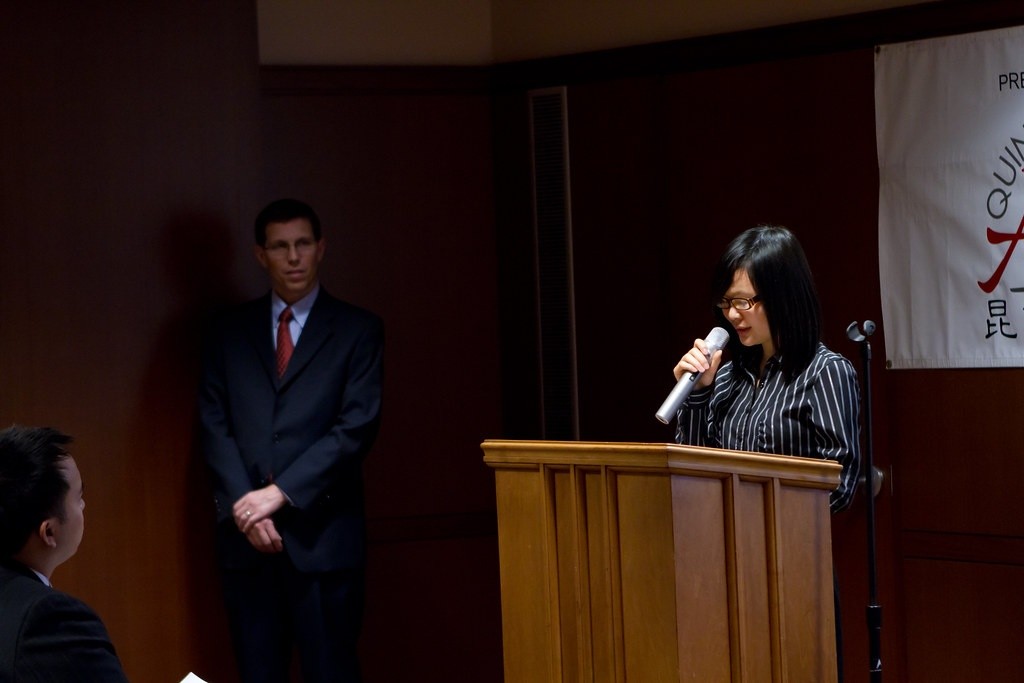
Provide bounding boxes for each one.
[655,327,729,424]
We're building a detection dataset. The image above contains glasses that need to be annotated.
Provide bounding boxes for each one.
[714,294,762,310]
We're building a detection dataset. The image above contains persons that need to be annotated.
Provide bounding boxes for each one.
[0,425,129,683]
[674,225,864,516]
[200,198,383,683]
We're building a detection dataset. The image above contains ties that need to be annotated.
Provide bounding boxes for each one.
[275,307,295,378]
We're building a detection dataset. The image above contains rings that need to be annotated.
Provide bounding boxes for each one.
[246,510,251,517]
